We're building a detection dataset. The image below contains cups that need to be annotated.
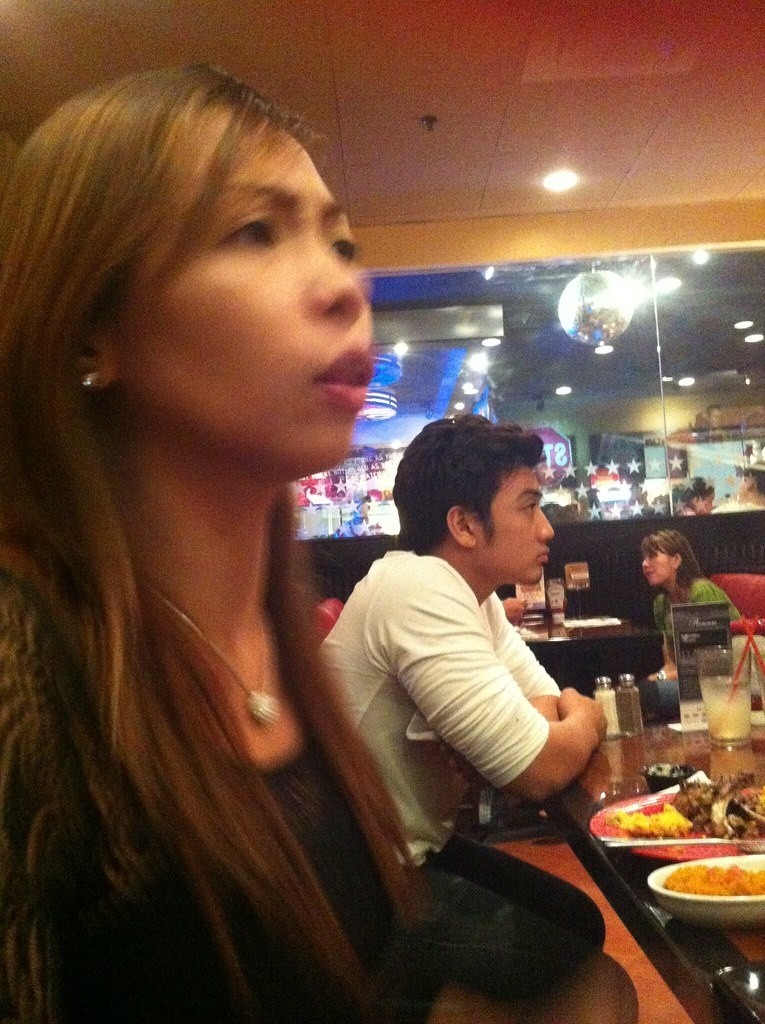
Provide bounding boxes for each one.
[694,644,752,747]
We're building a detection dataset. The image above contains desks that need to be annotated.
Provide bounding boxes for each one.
[543,722,765,1024]
[504,602,664,695]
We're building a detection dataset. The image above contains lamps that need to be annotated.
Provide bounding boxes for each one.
[353,353,403,424]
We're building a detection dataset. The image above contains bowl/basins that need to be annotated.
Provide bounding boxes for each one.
[640,765,696,792]
[647,855,765,930]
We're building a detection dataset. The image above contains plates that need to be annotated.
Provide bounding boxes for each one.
[589,788,765,862]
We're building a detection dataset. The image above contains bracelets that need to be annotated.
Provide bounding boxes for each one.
[656,668,671,681]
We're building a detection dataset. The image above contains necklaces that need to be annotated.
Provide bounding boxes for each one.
[145,583,279,727]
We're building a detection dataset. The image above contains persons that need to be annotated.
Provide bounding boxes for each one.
[305,553,352,638]
[318,411,640,1023]
[501,446,765,720]
[0,59,437,1024]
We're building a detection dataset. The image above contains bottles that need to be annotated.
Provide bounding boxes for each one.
[594,676,620,739]
[616,674,643,736]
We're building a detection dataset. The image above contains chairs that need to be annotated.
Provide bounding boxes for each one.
[707,569,765,636]
